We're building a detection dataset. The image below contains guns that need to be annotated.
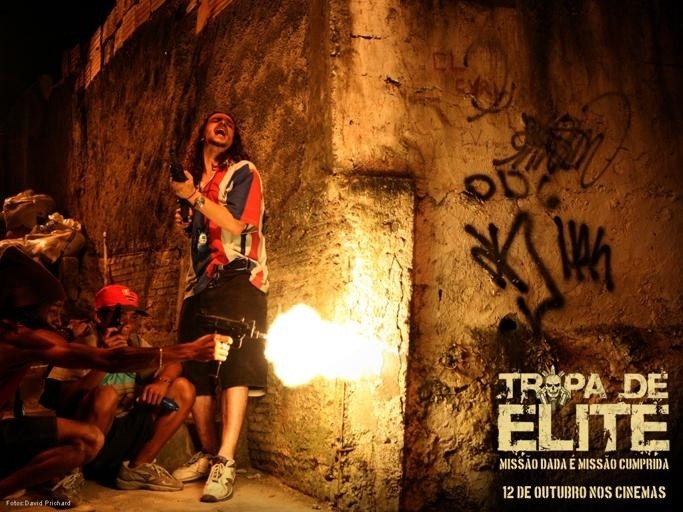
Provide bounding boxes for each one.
[110,302,124,336]
[202,310,270,379]
[169,148,193,235]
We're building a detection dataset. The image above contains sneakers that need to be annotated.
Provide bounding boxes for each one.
[170,449,216,482]
[37,470,86,510]
[199,455,237,503]
[114,460,185,492]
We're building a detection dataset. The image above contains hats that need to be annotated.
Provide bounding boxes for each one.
[90,283,150,319]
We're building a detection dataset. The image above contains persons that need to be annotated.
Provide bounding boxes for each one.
[1,239,233,507]
[169,110,268,500]
